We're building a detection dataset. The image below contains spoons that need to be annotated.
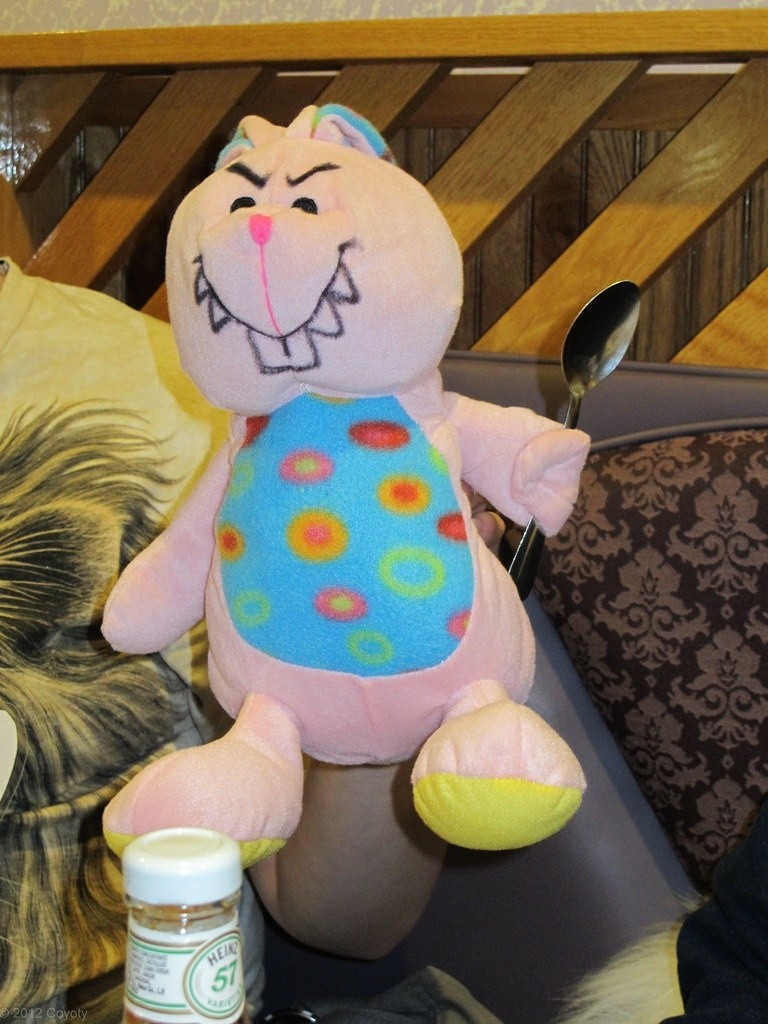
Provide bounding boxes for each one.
[505,280,642,600]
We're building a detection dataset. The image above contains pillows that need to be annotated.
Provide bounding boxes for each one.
[484,420,768,892]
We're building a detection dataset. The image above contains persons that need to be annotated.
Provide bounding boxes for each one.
[0,256,504,1023]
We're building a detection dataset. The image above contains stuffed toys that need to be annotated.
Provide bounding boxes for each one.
[98,104,594,874]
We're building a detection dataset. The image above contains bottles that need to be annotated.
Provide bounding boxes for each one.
[119,826,251,1024]
